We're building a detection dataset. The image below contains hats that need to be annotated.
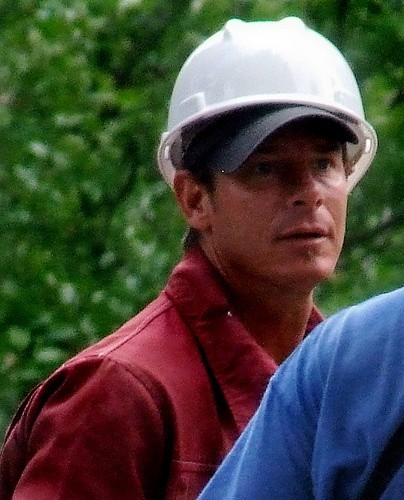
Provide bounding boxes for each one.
[179,102,359,175]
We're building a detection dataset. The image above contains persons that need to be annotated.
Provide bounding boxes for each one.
[0,17,379,500]
[196,286,404,500]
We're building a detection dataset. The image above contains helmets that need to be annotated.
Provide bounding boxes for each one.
[157,16,376,195]
